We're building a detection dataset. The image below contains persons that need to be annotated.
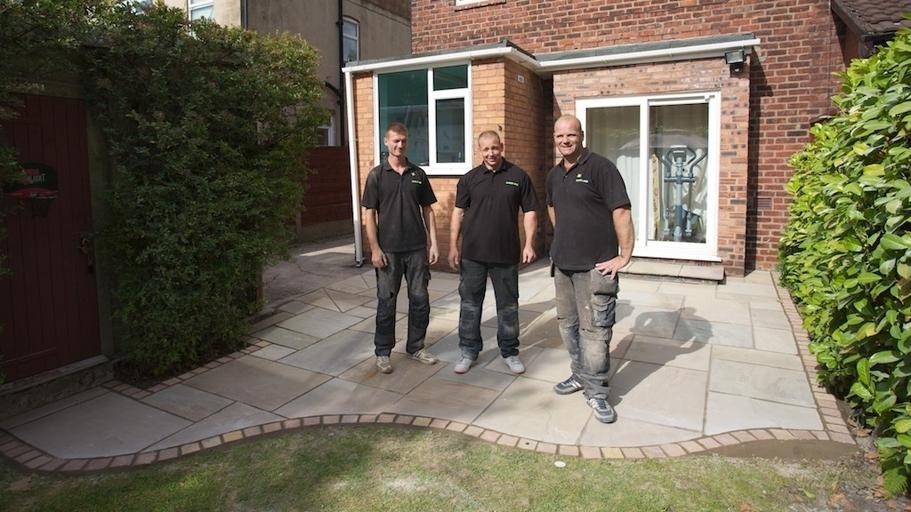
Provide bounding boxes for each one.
[544,114,635,423]
[447,130,541,374]
[360,121,440,374]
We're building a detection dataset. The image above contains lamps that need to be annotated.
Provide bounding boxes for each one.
[726,50,746,75]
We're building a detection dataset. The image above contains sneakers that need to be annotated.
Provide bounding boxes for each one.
[376,355,392,373]
[454,356,473,373]
[587,398,614,422]
[554,377,584,394]
[501,356,525,373]
[406,348,437,364]
